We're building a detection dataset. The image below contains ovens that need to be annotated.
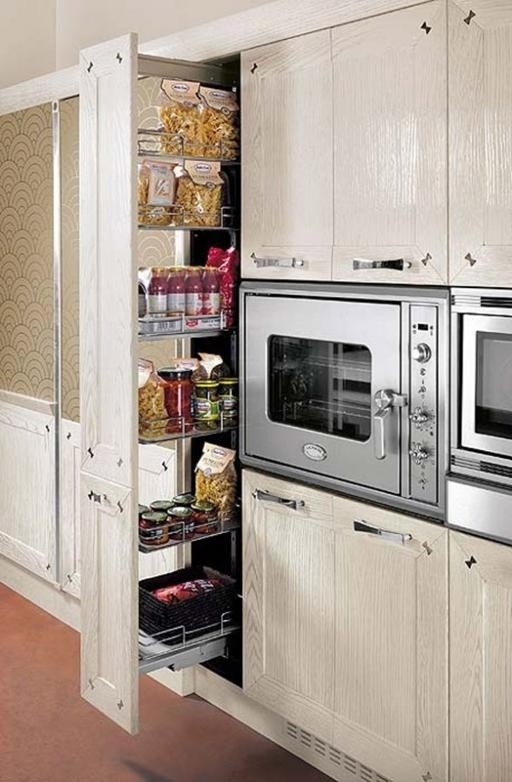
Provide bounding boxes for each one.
[446,287,512,547]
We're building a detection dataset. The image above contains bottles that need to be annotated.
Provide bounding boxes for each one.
[135,264,235,328]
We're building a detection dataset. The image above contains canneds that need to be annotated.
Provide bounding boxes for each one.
[156,366,193,434]
[139,494,213,545]
[194,380,225,421]
[218,376,238,422]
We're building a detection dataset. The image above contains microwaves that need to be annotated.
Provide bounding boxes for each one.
[238,280,450,525]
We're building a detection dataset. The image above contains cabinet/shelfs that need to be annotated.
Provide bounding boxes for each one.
[3,103,81,594]
[77,32,246,739]
[240,473,512,782]
[242,0,511,287]
